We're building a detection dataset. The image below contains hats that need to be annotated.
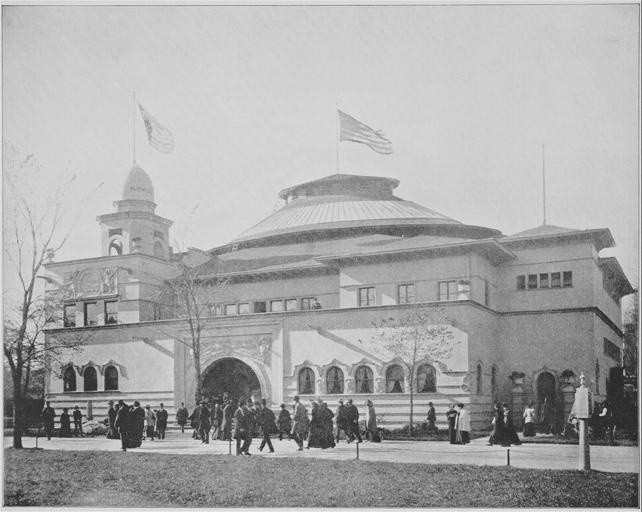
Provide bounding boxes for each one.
[133,401,140,407]
[199,401,206,405]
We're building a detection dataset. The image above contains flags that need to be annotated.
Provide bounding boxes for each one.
[338,109,391,154]
[136,101,176,155]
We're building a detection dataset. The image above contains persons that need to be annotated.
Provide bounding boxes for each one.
[365,399,382,442]
[484,395,612,448]
[452,402,467,444]
[188,393,362,455]
[447,402,458,442]
[60,407,71,438]
[42,400,57,441]
[426,400,435,431]
[311,297,322,310]
[73,404,85,438]
[107,400,168,451]
[176,401,189,433]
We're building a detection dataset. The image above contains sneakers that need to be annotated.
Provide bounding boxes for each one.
[257,447,262,452]
[239,450,244,455]
[266,450,275,454]
[244,452,254,456]
[296,447,303,451]
[304,446,311,450]
